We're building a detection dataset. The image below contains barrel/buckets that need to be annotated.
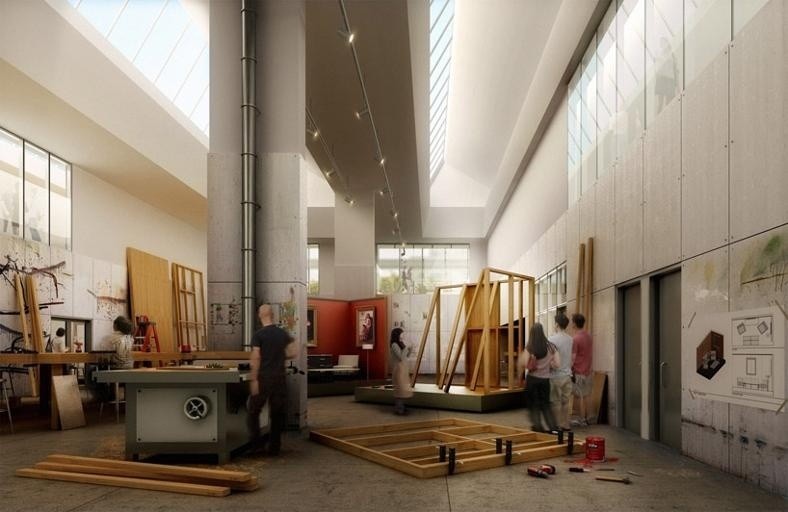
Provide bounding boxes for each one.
[585,437,606,464]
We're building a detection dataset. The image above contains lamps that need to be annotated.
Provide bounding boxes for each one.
[332,0,406,256]
[305,105,354,205]
[362,344,373,381]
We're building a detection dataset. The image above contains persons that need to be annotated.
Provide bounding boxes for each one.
[548,314,572,431]
[389,328,416,415]
[521,322,560,431]
[237,303,300,458]
[52,328,70,375]
[571,314,596,426]
[86,316,135,401]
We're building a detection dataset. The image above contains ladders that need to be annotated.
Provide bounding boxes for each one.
[133,322,163,369]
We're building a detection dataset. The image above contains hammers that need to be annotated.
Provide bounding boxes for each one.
[596,474,629,484]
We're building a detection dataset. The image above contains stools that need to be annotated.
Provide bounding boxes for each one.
[98,383,124,422]
[0,378,14,433]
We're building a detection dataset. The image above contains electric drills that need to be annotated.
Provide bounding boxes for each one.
[527,464,555,478]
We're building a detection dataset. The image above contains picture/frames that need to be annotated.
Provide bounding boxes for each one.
[306,305,318,349]
[355,305,375,348]
